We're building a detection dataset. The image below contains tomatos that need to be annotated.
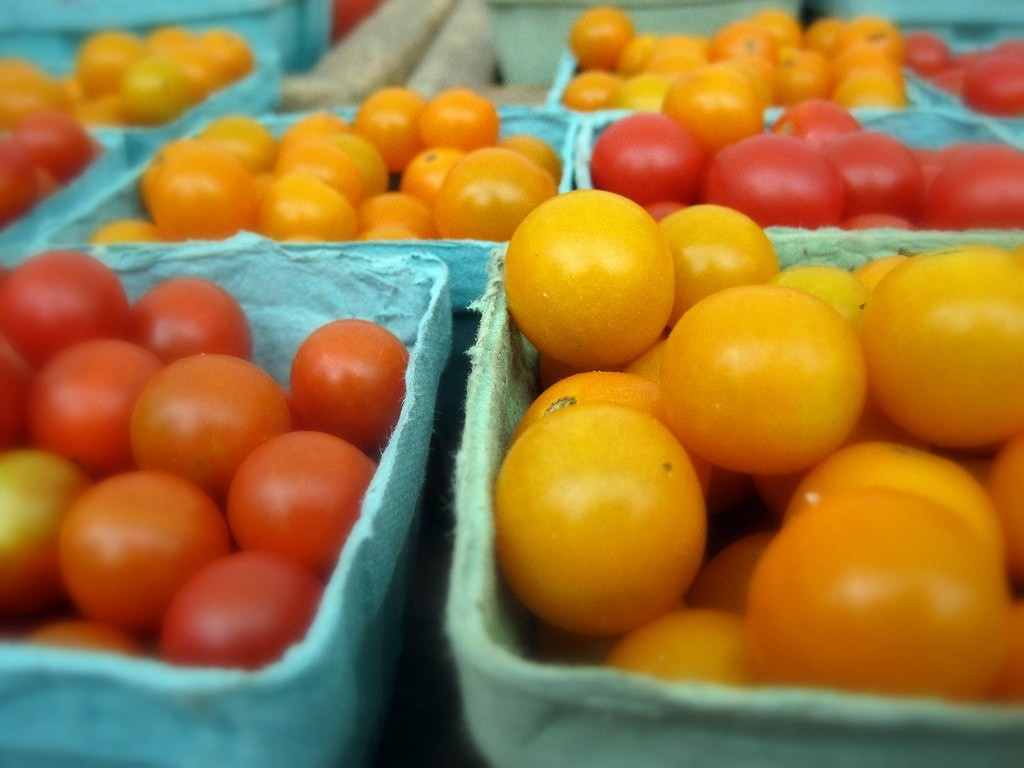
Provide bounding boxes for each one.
[0,249,405,669]
[501,189,1024,715]
[0,0,1024,244]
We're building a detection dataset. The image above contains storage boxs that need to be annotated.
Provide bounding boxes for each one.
[0,0,1023,768]
